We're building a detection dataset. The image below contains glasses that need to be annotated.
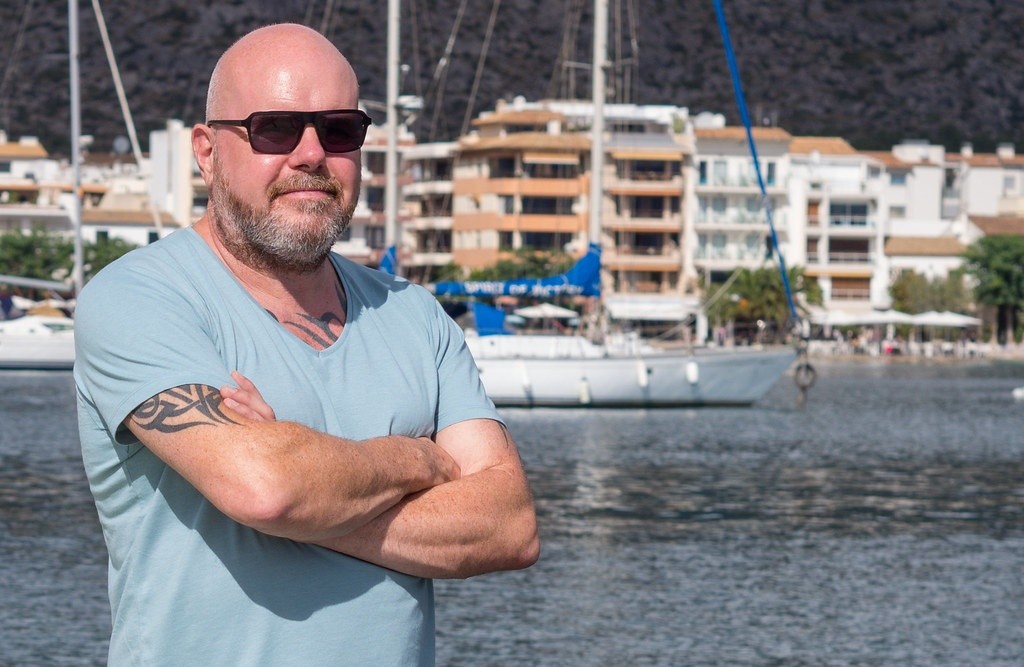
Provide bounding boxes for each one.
[206,109,374,154]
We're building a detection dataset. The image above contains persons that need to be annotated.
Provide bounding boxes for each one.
[73,24,540,666]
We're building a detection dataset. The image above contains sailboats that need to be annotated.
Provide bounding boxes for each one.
[0,1,164,369]
[377,0,809,408]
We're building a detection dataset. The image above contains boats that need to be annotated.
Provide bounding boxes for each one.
[785,309,990,376]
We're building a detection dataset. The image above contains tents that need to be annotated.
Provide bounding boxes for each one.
[813,306,983,357]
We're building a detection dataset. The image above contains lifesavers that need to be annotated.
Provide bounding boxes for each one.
[792,361,818,390]
